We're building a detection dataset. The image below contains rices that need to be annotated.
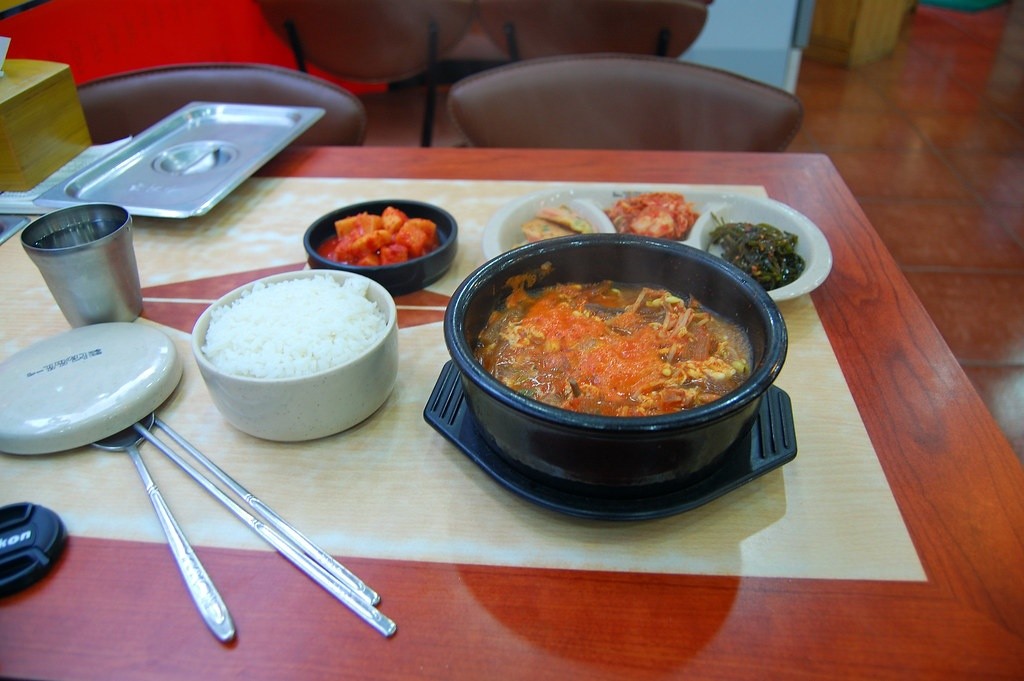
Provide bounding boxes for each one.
[201,273,387,379]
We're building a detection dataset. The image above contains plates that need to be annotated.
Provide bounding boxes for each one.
[423,359,796,521]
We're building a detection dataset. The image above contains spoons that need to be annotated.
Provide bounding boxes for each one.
[92,412,236,643]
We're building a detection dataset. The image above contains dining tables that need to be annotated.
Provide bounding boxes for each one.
[0,146,1024,681]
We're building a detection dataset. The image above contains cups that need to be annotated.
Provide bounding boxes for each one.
[21,203,143,329]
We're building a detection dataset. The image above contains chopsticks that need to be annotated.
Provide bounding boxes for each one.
[132,416,397,639]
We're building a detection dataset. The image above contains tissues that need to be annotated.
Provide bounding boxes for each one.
[0,38,92,193]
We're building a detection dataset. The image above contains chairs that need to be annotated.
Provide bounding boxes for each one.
[480,0,707,58]
[448,55,802,154]
[258,1,479,146]
[76,64,369,144]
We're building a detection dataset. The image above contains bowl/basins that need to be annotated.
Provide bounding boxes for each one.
[303,198,457,298]
[443,233,790,495]
[189,270,400,443]
